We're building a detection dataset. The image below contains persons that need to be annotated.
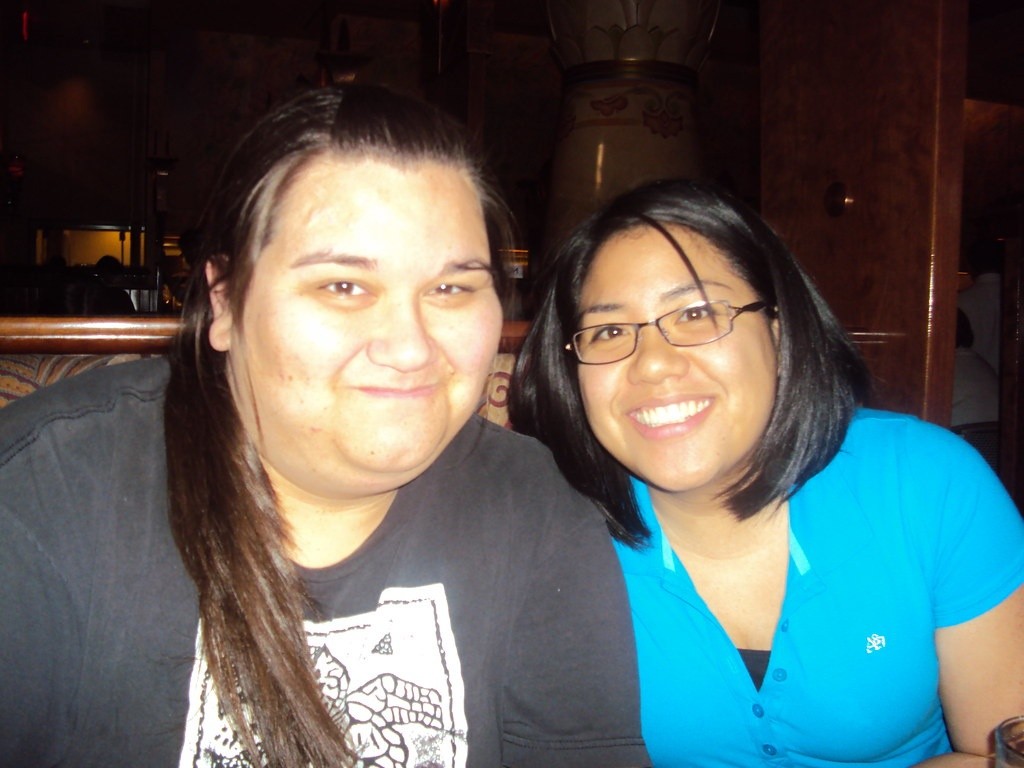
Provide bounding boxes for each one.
[2,84,653,767]
[504,177,1023,768]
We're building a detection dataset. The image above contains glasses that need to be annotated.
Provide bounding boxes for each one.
[566,299,769,365]
